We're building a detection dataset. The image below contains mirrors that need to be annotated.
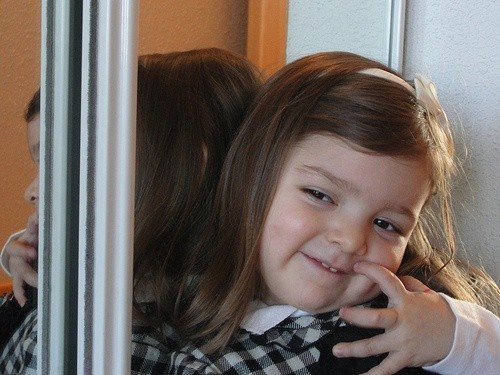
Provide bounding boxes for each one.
[78,0,406,332]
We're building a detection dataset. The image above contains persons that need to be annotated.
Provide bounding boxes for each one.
[128,46,273,375]
[0,88,41,374]
[156,51,499,375]
